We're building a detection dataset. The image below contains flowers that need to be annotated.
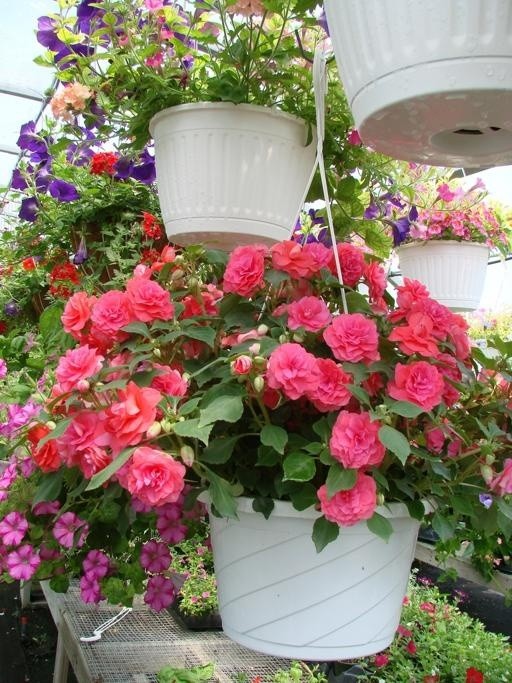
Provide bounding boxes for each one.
[0,0,511,682]
